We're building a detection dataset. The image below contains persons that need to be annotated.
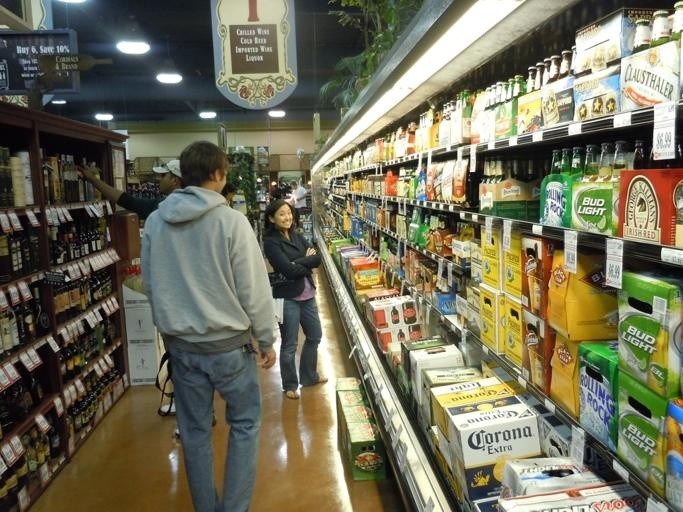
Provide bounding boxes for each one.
[220,183,237,202]
[141,139,279,512]
[254,176,307,227]
[76,157,217,438]
[262,197,329,400]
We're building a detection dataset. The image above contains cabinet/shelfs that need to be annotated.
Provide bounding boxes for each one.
[0,100,131,510]
[310,0,681,512]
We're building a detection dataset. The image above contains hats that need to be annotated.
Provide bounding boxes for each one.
[152,159,181,178]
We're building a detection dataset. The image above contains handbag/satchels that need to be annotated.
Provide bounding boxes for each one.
[268,270,296,287]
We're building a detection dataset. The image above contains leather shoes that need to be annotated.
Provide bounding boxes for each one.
[317,376,328,383]
[286,389,298,399]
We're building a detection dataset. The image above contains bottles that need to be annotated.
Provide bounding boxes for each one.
[343,194,378,223]
[647,416,683,512]
[645,308,670,400]
[612,139,627,170]
[433,268,465,294]
[0,146,106,210]
[55,317,118,385]
[407,324,422,341]
[479,155,544,184]
[647,138,666,169]
[0,288,50,357]
[65,361,124,442]
[548,148,559,175]
[401,302,416,325]
[0,215,40,283]
[571,145,581,176]
[390,307,399,325]
[584,143,596,173]
[541,57,549,84]
[482,74,523,110]
[549,54,560,81]
[345,174,387,195]
[365,88,477,166]
[343,215,379,252]
[0,366,45,436]
[666,135,683,169]
[648,10,670,47]
[557,49,570,77]
[402,207,453,260]
[396,329,404,342]
[670,0,683,41]
[0,417,63,512]
[123,264,144,293]
[524,65,534,91]
[560,146,571,178]
[43,207,110,266]
[631,139,646,169]
[377,235,400,270]
[48,262,115,325]
[629,17,650,55]
[534,62,543,90]
[569,44,577,74]
[599,141,610,171]
[423,213,449,230]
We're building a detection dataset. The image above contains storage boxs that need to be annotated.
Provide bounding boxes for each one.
[336,241,398,297]
[121,282,166,391]
[333,375,387,482]
[366,297,545,464]
